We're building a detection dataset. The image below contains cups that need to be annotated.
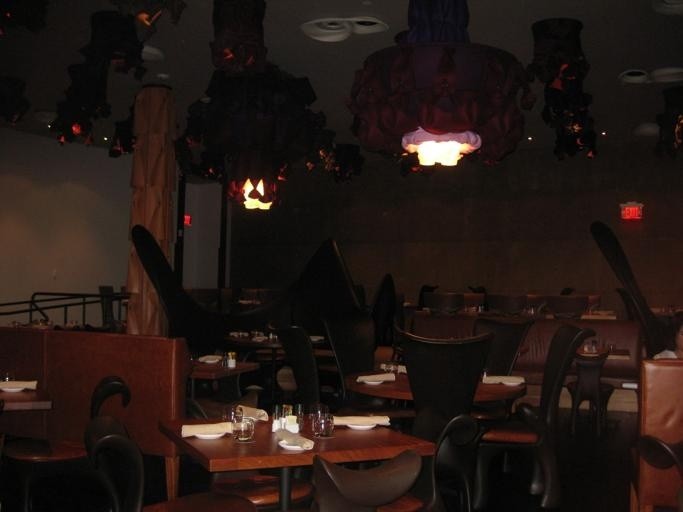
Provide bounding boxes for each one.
[299,402,335,439]
[222,405,254,442]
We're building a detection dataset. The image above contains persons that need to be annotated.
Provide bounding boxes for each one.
[652,311,683,359]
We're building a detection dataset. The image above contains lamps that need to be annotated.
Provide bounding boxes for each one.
[345,0,536,176]
[48,10,146,157]
[172,0,336,211]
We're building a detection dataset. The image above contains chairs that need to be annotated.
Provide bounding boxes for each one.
[0,285,683,512]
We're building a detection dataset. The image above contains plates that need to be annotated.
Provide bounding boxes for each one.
[364,380,384,385]
[346,423,377,430]
[3,388,26,393]
[278,439,314,450]
[501,382,521,386]
